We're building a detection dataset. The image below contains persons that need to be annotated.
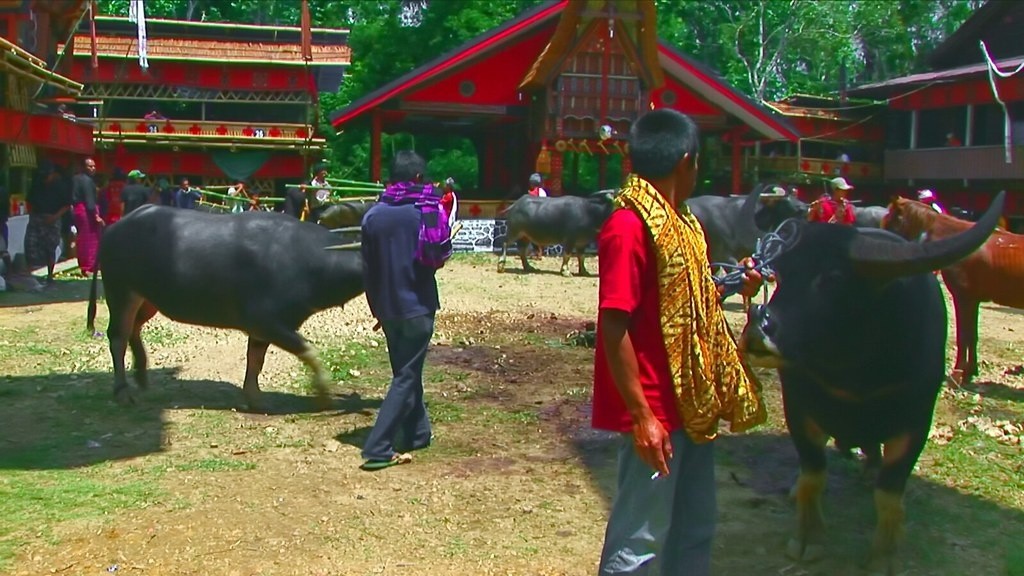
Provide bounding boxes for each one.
[357,149,454,468]
[144,111,170,120]
[772,132,962,278]
[20,156,333,288]
[438,174,459,227]
[528,172,547,259]
[590,106,768,576]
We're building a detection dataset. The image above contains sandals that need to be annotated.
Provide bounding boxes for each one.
[363,450,402,468]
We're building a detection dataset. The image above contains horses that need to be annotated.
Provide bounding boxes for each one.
[875,195,1024,394]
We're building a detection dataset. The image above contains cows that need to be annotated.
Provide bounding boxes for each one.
[86,204,367,418]
[760,189,1007,576]
[682,180,810,314]
[497,188,623,278]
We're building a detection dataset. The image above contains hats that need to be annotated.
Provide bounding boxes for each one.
[128,170,146,179]
[918,189,936,203]
[831,177,853,190]
